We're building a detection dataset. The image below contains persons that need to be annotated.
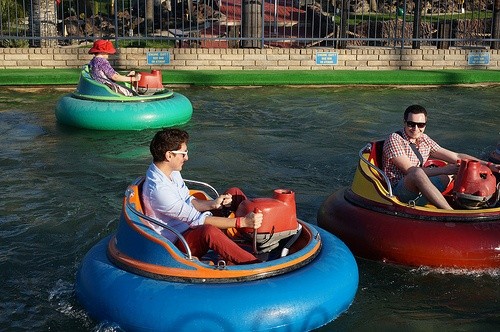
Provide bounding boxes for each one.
[142,128,263,264]
[382,105,500,211]
[89,40,141,97]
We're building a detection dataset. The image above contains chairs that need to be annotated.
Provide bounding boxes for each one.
[351,140,399,201]
[114,176,220,270]
[78,64,130,97]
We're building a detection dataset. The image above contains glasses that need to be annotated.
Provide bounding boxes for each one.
[171,149,189,158]
[406,120,426,129]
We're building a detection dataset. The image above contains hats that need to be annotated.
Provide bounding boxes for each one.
[88,40,116,54]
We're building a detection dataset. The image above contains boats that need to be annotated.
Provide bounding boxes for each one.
[317,138,500,271]
[55,64,193,131]
[76,175,360,332]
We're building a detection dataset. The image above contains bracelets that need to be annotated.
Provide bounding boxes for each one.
[236,217,240,228]
[487,162,491,167]
[131,77,132,82]
